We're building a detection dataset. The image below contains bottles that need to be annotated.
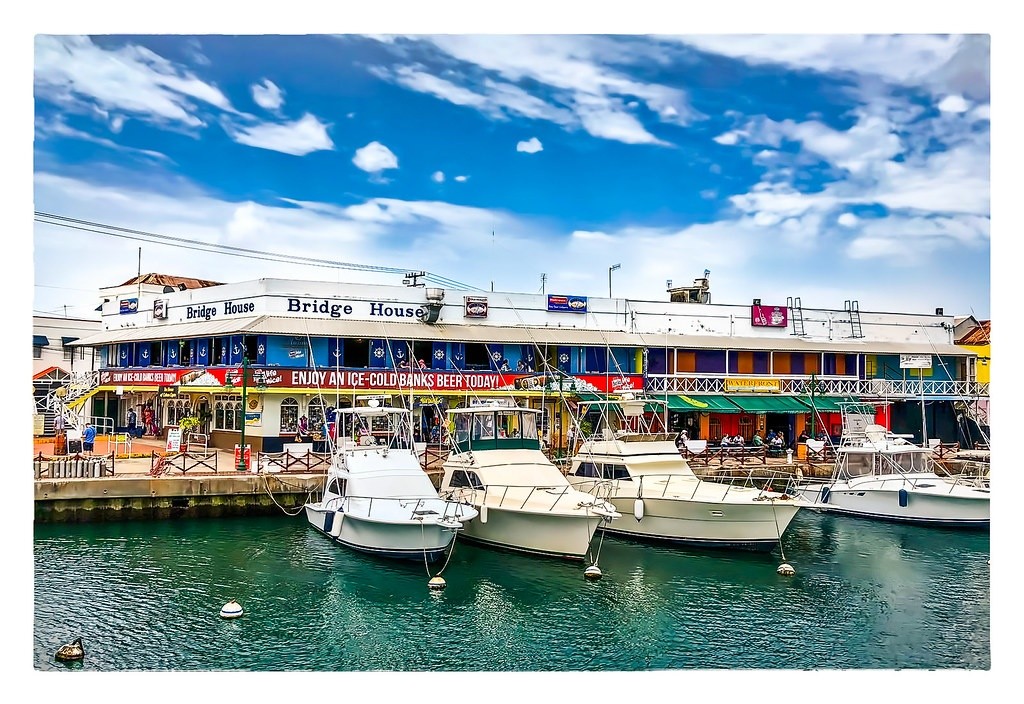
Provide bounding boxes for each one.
[514,375,556,390]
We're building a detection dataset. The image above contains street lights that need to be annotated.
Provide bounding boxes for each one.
[225,356,266,472]
[55,387,66,436]
[800,370,826,442]
[543,370,578,460]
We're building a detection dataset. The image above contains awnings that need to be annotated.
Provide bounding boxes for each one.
[575,394,877,414]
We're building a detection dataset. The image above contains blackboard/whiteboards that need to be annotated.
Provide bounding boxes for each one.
[166,429,181,452]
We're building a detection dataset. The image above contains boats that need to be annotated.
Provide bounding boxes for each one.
[564,390,838,543]
[304,398,480,564]
[438,399,617,562]
[788,401,990,525]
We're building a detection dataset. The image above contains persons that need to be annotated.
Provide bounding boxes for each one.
[543,432,548,447]
[127,408,136,438]
[501,359,512,371]
[733,434,744,451]
[511,428,519,437]
[753,429,787,457]
[798,429,830,455]
[566,425,575,457]
[517,360,524,371]
[397,359,426,369]
[53,413,64,436]
[681,430,690,457]
[720,433,732,456]
[81,423,97,458]
